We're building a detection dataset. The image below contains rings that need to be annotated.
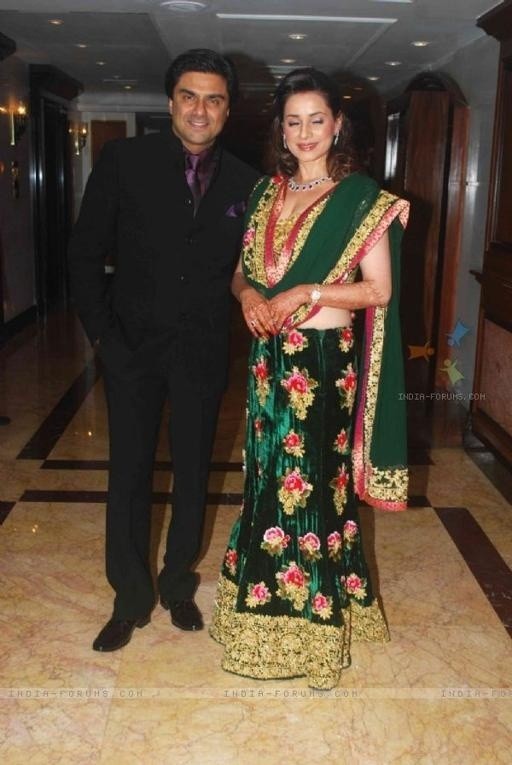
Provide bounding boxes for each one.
[250,318,257,328]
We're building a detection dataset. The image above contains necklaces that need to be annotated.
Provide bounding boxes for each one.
[288,173,332,193]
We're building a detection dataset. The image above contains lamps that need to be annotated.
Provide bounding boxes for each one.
[75,125,87,156]
[9,101,29,147]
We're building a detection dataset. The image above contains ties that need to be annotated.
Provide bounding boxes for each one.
[185,153,203,216]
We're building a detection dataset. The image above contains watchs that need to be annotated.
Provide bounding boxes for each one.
[309,281,321,308]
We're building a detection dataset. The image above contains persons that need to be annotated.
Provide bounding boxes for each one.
[207,67,411,691]
[61,48,268,654]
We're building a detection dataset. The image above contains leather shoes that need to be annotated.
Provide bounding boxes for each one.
[170,598,203,631]
[92,609,152,652]
